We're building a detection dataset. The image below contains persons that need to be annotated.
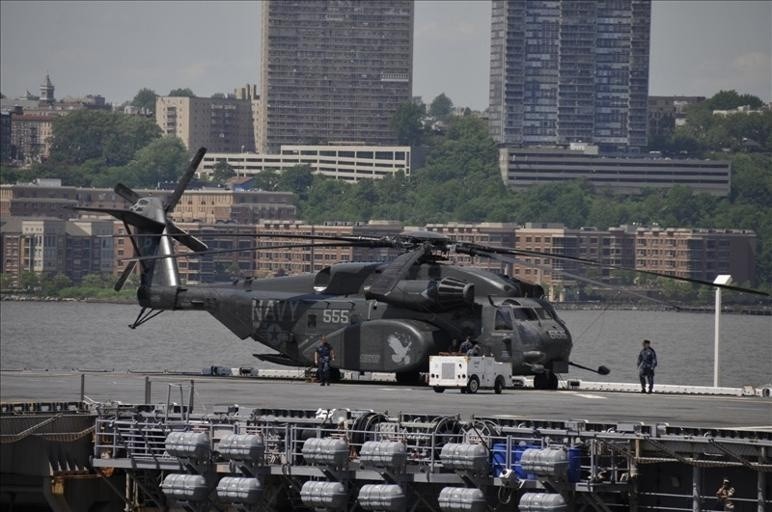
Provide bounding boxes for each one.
[448,335,480,356]
[314,335,336,386]
[636,340,657,393]
[715,479,736,512]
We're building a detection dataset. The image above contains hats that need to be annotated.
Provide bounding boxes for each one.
[644,340,650,343]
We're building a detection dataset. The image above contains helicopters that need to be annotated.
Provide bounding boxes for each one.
[61,146,771,390]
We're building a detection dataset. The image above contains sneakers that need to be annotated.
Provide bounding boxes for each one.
[641,389,652,393]
[320,381,330,386]
